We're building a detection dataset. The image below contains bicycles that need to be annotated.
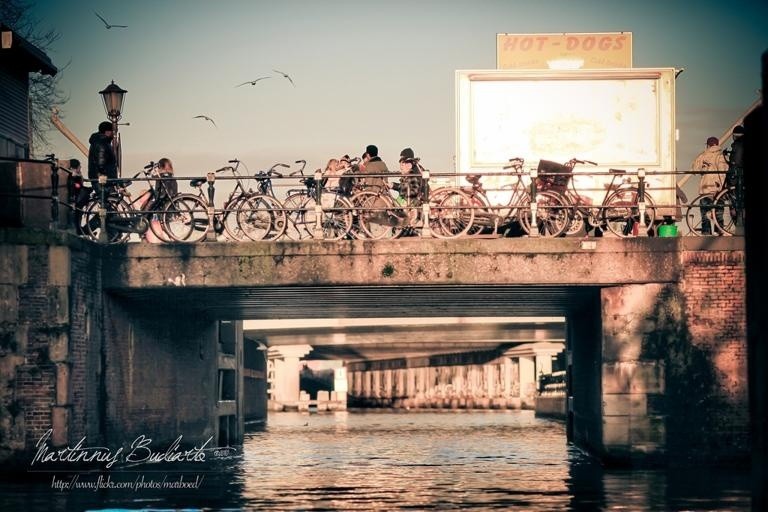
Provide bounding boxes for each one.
[65,157,744,237]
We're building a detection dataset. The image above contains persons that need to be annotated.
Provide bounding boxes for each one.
[74,121,124,242]
[692,136,731,236]
[677,183,688,223]
[152,157,180,219]
[69,154,86,238]
[321,142,428,239]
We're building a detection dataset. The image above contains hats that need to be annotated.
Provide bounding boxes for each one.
[704,136,719,146]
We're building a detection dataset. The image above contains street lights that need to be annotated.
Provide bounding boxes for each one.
[99,78,124,159]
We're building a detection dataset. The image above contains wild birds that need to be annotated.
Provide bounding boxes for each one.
[94,11,128,29]
[273,70,296,88]
[193,115,216,127]
[236,76,271,87]
